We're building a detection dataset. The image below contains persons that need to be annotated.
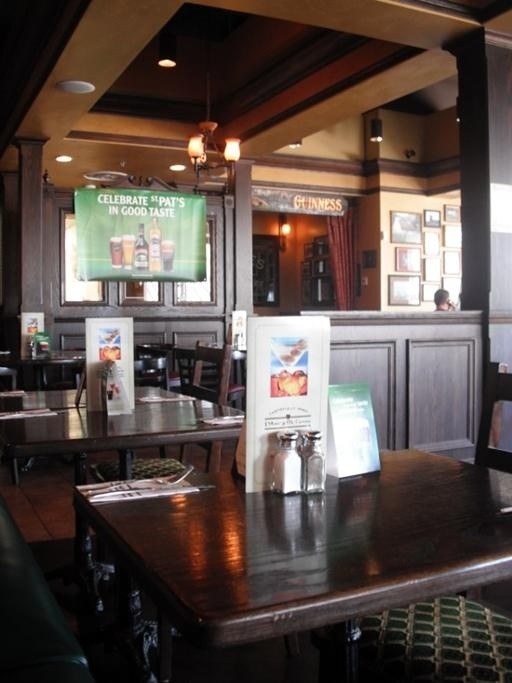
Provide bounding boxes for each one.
[433,290,455,311]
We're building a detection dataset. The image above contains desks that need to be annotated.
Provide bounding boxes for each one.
[1,386,246,562]
[72,447,512,683]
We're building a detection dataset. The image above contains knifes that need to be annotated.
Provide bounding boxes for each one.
[87,483,217,499]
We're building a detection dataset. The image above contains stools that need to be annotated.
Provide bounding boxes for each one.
[79,451,188,482]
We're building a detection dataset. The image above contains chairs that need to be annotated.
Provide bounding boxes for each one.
[474,359,511,478]
[131,339,234,474]
[2,334,247,386]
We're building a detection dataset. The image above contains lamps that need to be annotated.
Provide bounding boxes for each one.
[369,108,383,144]
[188,29,241,196]
[277,213,290,252]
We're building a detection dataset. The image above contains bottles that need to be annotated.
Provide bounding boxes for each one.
[303,492,330,553]
[136,216,162,271]
[272,431,300,494]
[298,430,327,494]
[271,495,303,557]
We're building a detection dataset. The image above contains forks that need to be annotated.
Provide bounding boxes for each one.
[79,465,199,492]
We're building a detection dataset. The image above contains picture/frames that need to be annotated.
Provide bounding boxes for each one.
[361,248,378,269]
[387,200,462,310]
[252,233,280,306]
[298,234,335,305]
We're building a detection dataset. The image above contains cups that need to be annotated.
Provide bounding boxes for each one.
[162,242,174,271]
[109,235,135,270]
